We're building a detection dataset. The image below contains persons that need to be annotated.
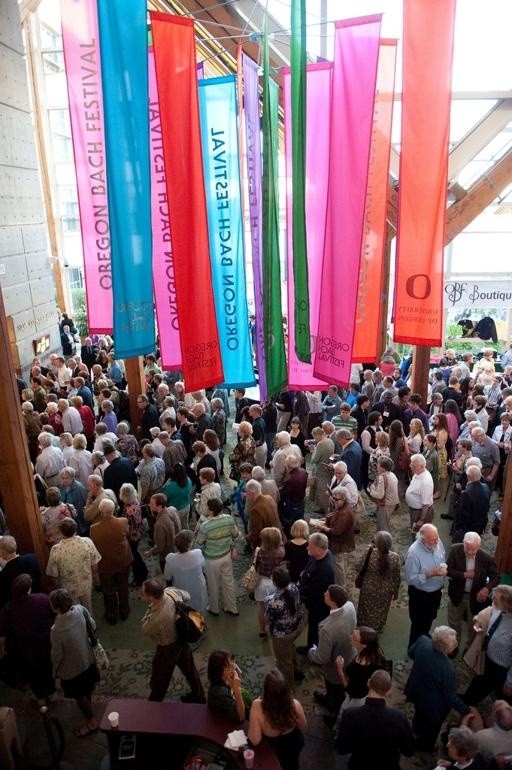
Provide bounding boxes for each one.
[0,311,509,768]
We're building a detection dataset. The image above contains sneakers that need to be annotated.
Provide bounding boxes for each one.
[440,513,453,520]
[296,645,309,656]
[313,691,326,701]
[180,693,207,704]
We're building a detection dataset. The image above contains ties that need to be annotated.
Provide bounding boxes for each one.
[480,611,503,651]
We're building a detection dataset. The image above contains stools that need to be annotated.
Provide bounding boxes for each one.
[0,708,23,769]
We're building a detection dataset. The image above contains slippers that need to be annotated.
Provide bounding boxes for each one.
[73,724,99,738]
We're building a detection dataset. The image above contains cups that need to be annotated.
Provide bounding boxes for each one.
[243,749,255,770]
[107,712,120,727]
[440,562,450,574]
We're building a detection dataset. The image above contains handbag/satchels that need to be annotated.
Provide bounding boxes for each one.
[174,600,206,644]
[355,569,364,589]
[141,505,156,528]
[370,495,386,507]
[91,641,110,676]
[240,564,261,591]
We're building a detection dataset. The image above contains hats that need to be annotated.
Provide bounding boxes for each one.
[149,427,160,437]
[101,445,116,457]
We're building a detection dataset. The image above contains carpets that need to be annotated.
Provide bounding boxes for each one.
[90,476,454,608]
[92,648,494,770]
[476,490,505,563]
